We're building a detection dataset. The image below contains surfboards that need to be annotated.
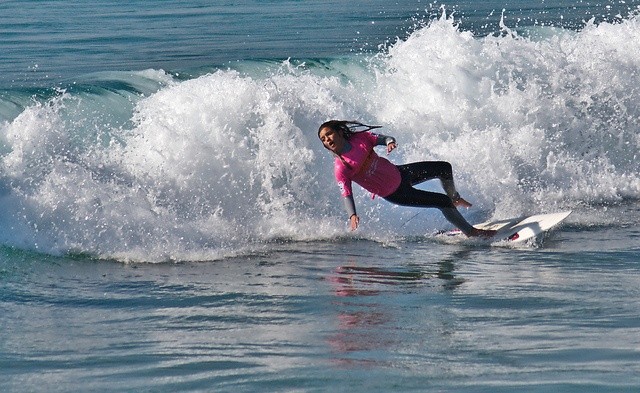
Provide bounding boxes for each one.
[445,211,572,246]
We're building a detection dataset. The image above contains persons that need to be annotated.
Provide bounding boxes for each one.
[318,120,496,240]
